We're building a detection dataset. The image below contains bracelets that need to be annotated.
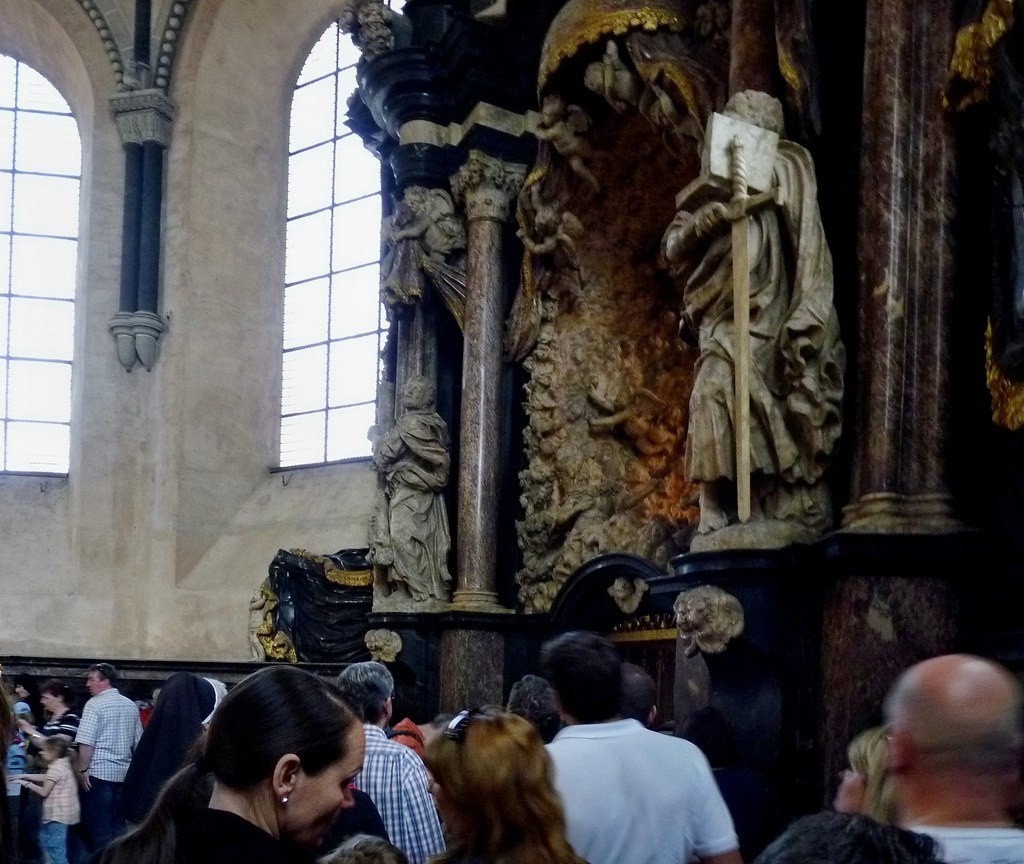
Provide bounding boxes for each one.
[25,782,32,788]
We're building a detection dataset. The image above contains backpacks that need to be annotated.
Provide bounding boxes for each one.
[6,744,26,779]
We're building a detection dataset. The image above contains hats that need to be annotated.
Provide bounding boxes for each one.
[110,670,229,825]
[13,702,31,714]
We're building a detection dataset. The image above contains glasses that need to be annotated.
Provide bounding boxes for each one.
[444,704,481,744]
[388,691,396,702]
[96,663,107,676]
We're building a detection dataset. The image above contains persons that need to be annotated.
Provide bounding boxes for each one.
[590,375,676,458]
[757,654,1024,864]
[367,373,451,601]
[0,631,740,864]
[518,181,588,290]
[584,40,656,132]
[393,185,465,263]
[664,91,845,533]
[524,94,615,191]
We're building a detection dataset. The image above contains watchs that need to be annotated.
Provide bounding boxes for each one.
[78,767,89,774]
